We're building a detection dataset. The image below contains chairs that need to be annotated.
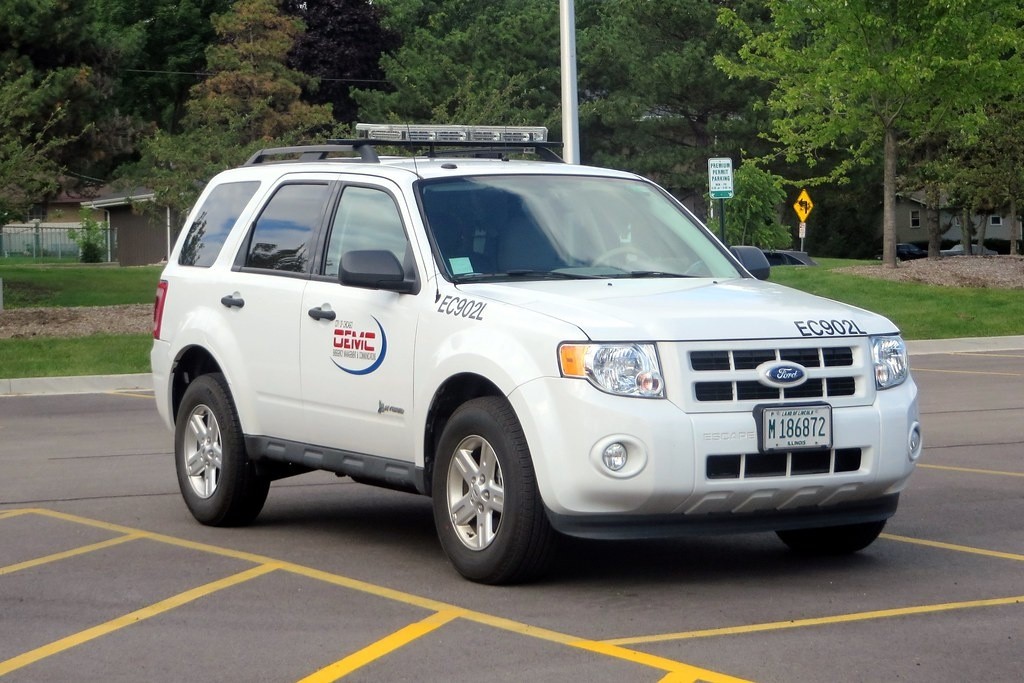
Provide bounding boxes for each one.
[494,217,559,272]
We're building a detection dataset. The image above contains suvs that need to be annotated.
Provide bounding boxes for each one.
[150,124,921,582]
